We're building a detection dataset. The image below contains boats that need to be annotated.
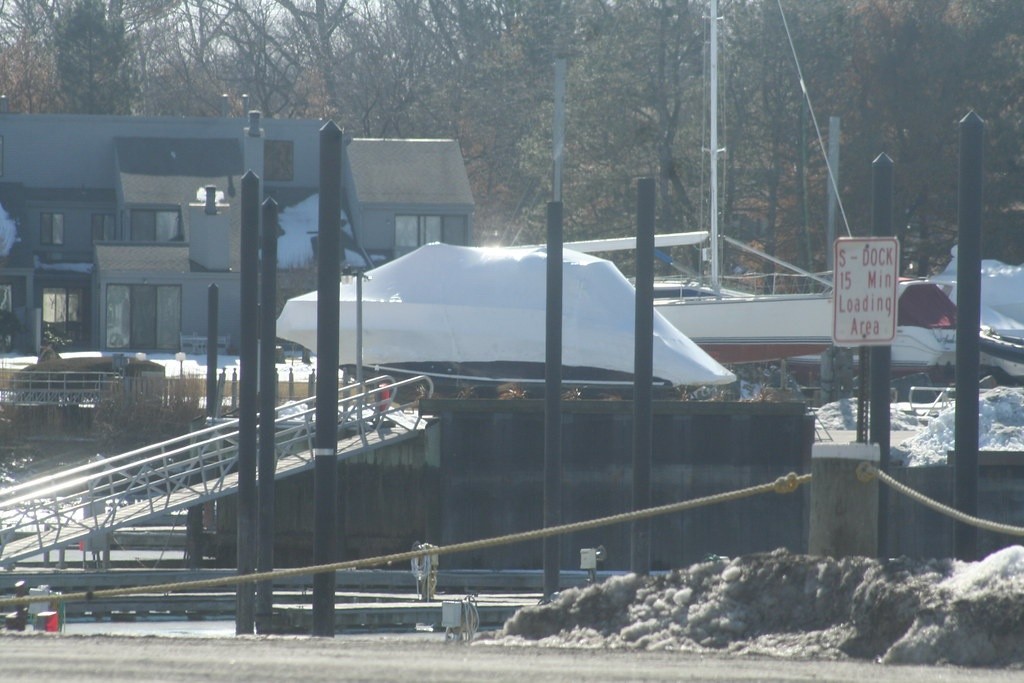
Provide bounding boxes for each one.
[270,242,737,408]
[554,0,958,378]
